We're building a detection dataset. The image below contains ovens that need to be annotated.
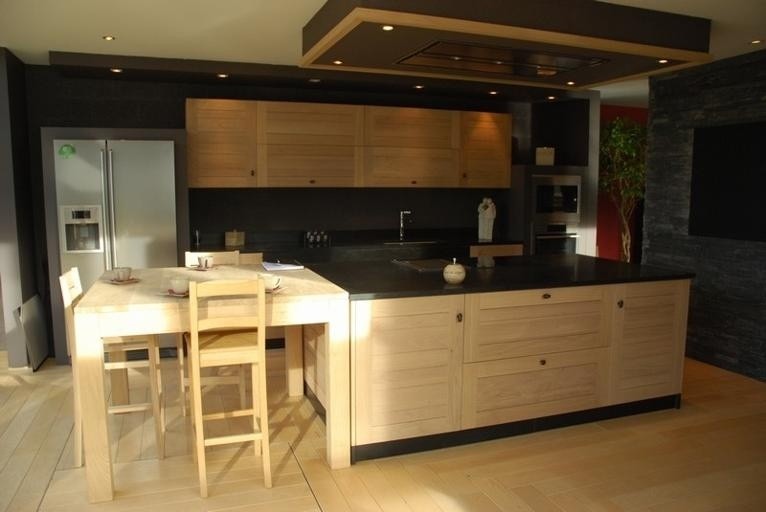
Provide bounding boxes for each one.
[530,175,581,255]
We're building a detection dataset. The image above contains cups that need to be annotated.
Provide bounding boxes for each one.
[257,273,281,291]
[113,267,131,281]
[198,255,214,268]
[171,275,189,295]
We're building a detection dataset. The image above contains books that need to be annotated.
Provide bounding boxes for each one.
[262,259,304,270]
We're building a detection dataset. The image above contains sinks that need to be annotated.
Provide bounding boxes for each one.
[384,239,435,245]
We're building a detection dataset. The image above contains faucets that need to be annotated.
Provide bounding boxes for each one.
[399,209,411,238]
